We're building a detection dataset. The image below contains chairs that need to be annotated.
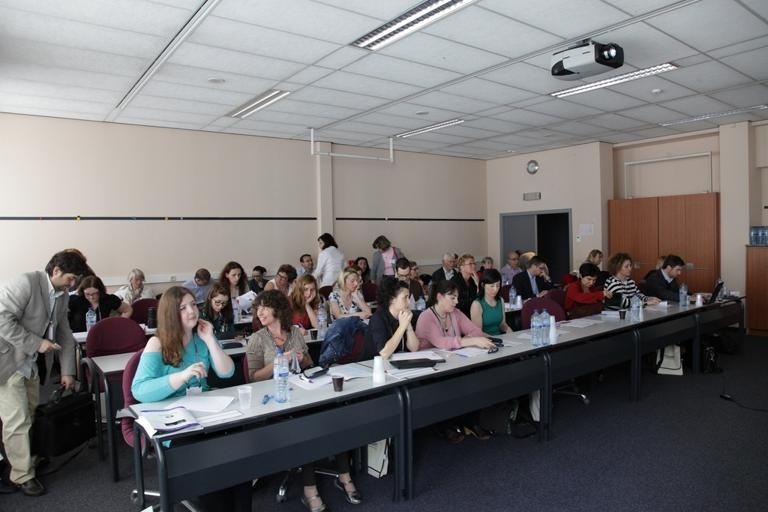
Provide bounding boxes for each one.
[521,296,565,330]
[84,317,146,452]
[121,346,201,510]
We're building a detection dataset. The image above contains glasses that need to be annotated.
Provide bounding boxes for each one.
[212,298,228,306]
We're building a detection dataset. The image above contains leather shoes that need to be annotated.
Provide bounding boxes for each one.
[437,424,491,443]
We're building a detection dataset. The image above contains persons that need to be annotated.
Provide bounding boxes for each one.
[312,233,346,303]
[63,254,421,512]
[0,247,76,497]
[415,250,688,443]
[370,235,406,286]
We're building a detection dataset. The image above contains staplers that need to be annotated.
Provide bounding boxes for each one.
[488,337,504,347]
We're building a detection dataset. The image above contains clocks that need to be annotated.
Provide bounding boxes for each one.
[527,160,538,174]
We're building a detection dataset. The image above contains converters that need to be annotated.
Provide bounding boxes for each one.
[720,394,731,399]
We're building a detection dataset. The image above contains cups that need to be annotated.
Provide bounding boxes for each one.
[332,375,344,392]
[620,310,627,319]
[374,356,384,373]
[696,295,703,306]
[550,315,556,329]
[516,295,523,309]
[310,329,318,340]
[238,385,252,410]
[687,295,690,306]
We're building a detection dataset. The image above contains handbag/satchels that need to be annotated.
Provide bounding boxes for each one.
[367,438,391,479]
[699,344,724,374]
[29,383,96,457]
[656,344,684,376]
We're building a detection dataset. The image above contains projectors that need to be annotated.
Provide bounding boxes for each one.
[551,43,624,81]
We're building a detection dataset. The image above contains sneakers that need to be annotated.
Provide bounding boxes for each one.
[16,477,46,497]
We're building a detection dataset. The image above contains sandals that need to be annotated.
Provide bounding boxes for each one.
[300,492,330,512]
[334,476,363,505]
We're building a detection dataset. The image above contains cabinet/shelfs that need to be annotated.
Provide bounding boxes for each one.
[744,244,768,336]
[608,192,720,293]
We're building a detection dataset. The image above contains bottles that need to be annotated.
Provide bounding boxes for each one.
[530,307,550,346]
[86,307,97,334]
[509,285,516,309]
[231,296,241,323]
[751,226,768,244]
[273,348,289,403]
[317,304,328,339]
[417,296,426,310]
[679,285,687,306]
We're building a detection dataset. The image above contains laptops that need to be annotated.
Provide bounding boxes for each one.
[690,282,723,304]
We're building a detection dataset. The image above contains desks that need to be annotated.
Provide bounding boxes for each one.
[668,292,743,370]
[358,336,551,501]
[73,288,526,384]
[127,363,409,511]
[496,317,639,439]
[589,301,698,401]
[91,337,249,484]
